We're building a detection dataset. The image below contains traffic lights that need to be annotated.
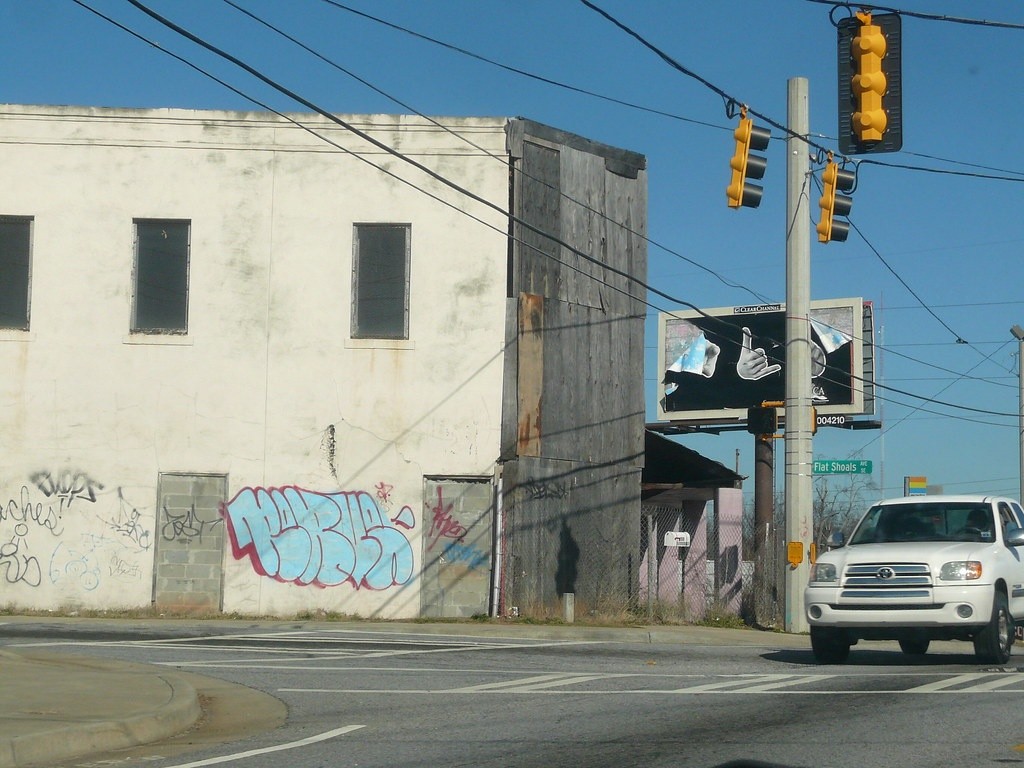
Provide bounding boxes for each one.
[817,163,856,245]
[726,118,771,208]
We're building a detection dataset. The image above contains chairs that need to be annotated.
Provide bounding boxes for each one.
[895,517,924,538]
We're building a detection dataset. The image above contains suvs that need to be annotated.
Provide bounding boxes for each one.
[803,495,1024,665]
